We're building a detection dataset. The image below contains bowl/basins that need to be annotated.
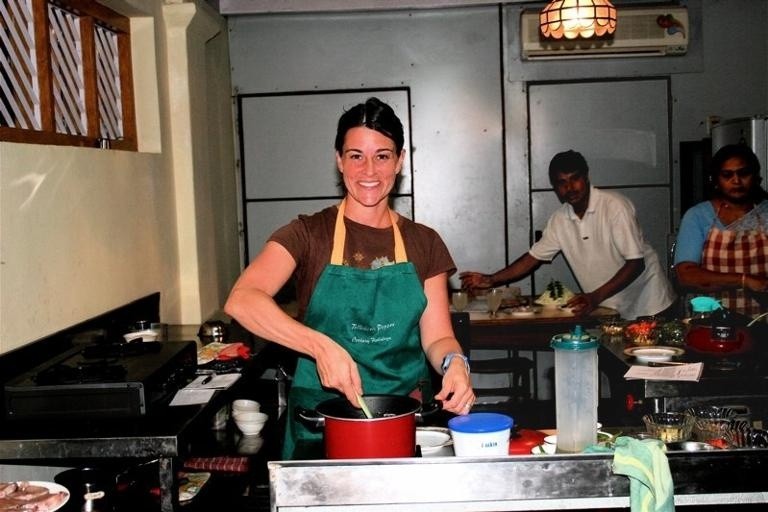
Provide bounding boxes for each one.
[237,413,267,434]
[234,400,259,419]
[642,412,696,445]
[664,442,721,452]
[687,405,736,430]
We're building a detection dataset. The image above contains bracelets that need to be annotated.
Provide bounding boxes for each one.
[742,274,745,289]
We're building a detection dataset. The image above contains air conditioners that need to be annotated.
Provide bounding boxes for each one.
[519,3,689,61]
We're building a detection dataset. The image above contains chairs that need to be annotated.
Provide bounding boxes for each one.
[468,349,535,405]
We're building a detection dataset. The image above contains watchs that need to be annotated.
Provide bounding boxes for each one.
[440,352,470,373]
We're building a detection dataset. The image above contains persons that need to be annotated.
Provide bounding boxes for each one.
[459,149,684,397]
[222,97,478,461]
[675,144,768,321]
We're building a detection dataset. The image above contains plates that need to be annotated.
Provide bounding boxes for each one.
[414,426,454,457]
[0,479,72,512]
[624,345,684,364]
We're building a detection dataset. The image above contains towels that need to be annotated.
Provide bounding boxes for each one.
[610,435,677,512]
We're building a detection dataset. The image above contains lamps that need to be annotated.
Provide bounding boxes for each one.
[540,0,619,45]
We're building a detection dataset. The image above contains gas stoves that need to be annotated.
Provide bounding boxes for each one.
[1,337,198,417]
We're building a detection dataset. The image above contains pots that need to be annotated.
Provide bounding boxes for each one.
[298,394,442,458]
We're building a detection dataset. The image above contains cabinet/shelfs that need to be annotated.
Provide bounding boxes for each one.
[586,317,768,425]
[0,325,297,512]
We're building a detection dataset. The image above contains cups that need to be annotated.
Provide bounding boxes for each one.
[484,289,503,320]
[452,292,468,315]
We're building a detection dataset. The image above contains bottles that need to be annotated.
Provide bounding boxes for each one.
[550,324,598,452]
